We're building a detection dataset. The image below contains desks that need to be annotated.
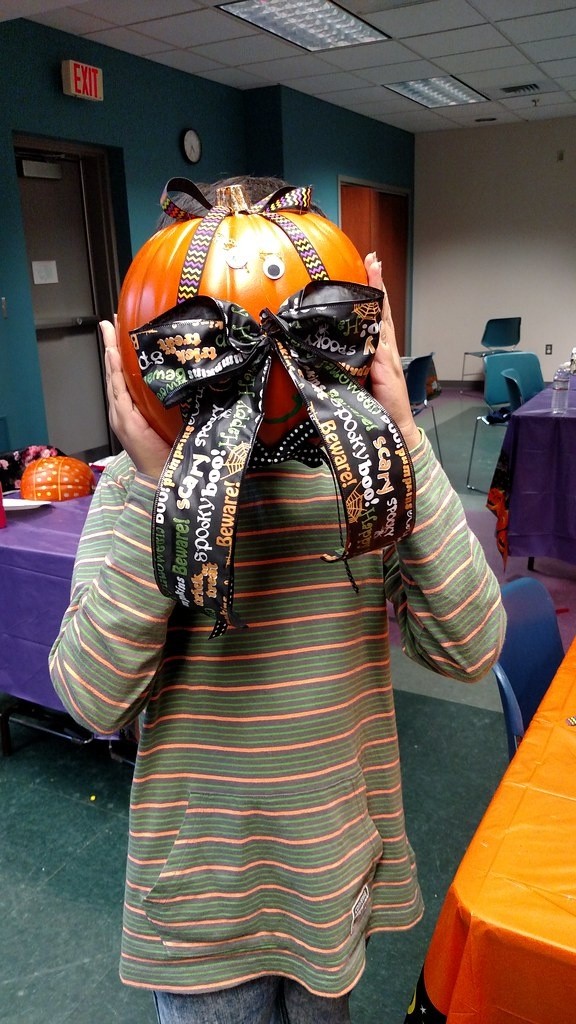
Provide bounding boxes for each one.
[400,633,575,1024]
[487,367,576,573]
[0,468,138,777]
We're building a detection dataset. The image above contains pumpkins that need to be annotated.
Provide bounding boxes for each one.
[19,456,102,501]
[116,184,372,447]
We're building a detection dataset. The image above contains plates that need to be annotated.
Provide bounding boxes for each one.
[2,498,51,510]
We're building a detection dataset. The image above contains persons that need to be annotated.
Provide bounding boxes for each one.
[48,175,507,1024]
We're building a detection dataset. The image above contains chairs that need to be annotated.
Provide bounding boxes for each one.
[466,351,546,495]
[459,316,522,400]
[402,352,444,471]
[500,367,524,415]
[477,575,566,769]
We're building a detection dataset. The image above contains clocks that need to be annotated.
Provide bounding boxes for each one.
[181,129,203,165]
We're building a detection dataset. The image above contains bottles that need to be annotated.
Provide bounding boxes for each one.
[0,481,7,529]
[570,348,576,375]
[551,364,570,413]
